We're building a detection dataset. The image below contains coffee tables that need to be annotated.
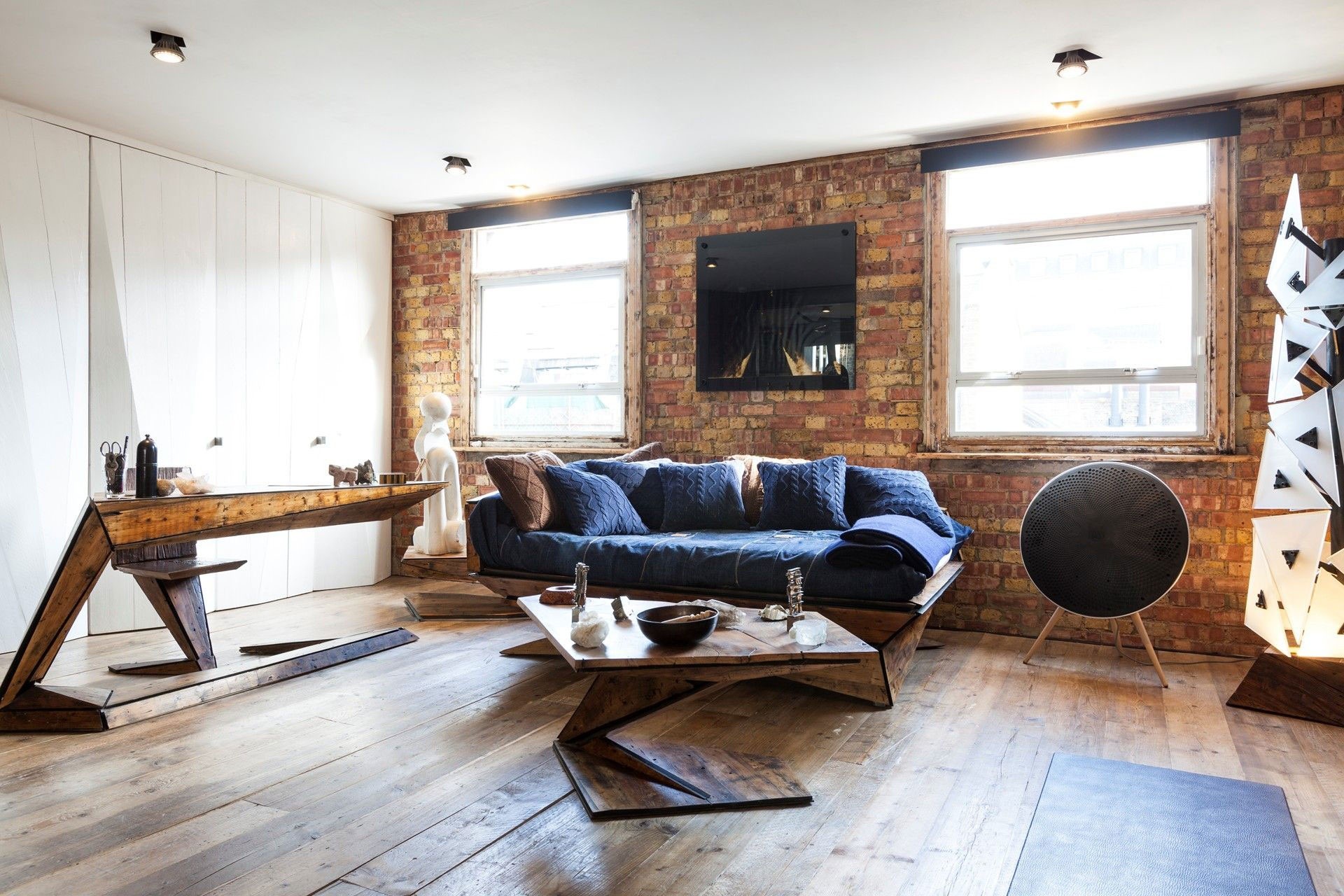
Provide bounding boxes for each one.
[517,595,879,821]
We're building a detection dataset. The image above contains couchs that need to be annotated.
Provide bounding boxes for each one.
[470,495,975,708]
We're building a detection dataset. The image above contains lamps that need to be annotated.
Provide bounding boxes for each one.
[445,157,466,176]
[1056,51,1088,78]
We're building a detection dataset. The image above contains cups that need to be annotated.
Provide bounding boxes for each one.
[105,475,124,499]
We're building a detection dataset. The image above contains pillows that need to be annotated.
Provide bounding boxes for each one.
[542,464,650,536]
[570,442,955,538]
[484,450,564,530]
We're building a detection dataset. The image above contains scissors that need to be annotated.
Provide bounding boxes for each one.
[100,442,122,484]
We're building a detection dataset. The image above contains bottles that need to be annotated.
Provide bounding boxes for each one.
[135,434,158,498]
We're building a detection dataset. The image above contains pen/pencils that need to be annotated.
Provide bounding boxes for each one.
[124,436,129,454]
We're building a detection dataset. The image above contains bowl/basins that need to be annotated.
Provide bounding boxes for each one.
[637,605,720,647]
[792,619,826,646]
[380,474,405,484]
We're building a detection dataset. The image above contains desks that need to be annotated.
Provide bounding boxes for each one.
[0,481,452,734]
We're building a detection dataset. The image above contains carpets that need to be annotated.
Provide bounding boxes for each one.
[1006,753,1318,896]
